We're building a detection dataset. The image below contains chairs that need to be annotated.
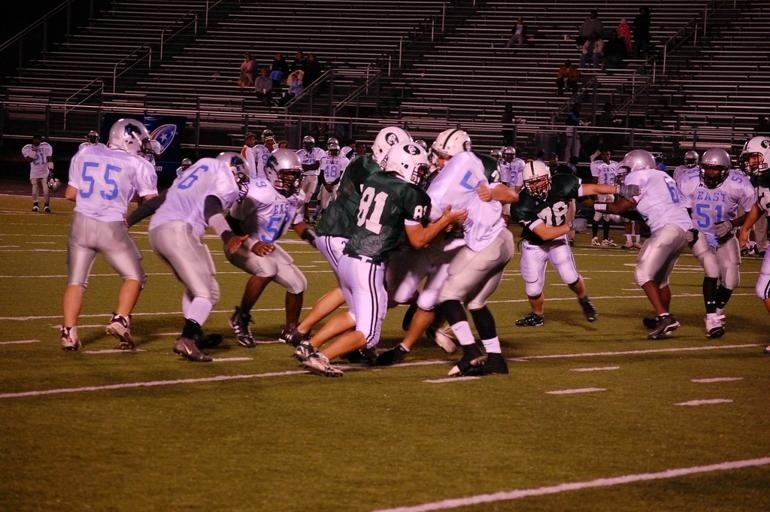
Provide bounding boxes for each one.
[515,310,545,327]
[591,236,618,247]
[642,314,681,340]
[762,345,770,353]
[61,325,83,350]
[277,323,408,377]
[105,312,137,350]
[402,305,509,377]
[580,295,599,321]
[229,306,257,347]
[703,310,726,338]
[33,203,51,212]
[172,333,223,362]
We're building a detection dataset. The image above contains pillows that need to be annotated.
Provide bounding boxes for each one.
[738,136,770,177]
[215,152,251,204]
[326,137,341,156]
[263,148,304,198]
[48,177,62,191]
[181,158,192,170]
[684,150,699,167]
[86,130,100,143]
[614,149,657,185]
[371,126,473,186]
[110,118,154,162]
[522,161,553,202]
[262,129,274,141]
[502,147,516,163]
[701,148,732,189]
[302,136,316,151]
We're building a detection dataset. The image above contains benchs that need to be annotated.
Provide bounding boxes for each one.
[0,0,770,163]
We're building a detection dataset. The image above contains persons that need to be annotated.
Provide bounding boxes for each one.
[738,136,768,303]
[686,148,759,338]
[59,119,159,351]
[500,103,522,147]
[505,15,528,52]
[78,130,100,151]
[290,125,515,378]
[554,7,653,115]
[510,159,641,326]
[491,114,757,256]
[240,128,368,232]
[235,48,327,114]
[148,152,250,362]
[137,124,177,209]
[224,148,318,347]
[581,149,694,339]
[22,133,54,213]
[177,158,192,176]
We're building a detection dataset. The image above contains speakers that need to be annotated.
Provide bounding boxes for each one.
[621,234,642,249]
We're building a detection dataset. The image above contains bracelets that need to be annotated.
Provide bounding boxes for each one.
[125,220,129,227]
